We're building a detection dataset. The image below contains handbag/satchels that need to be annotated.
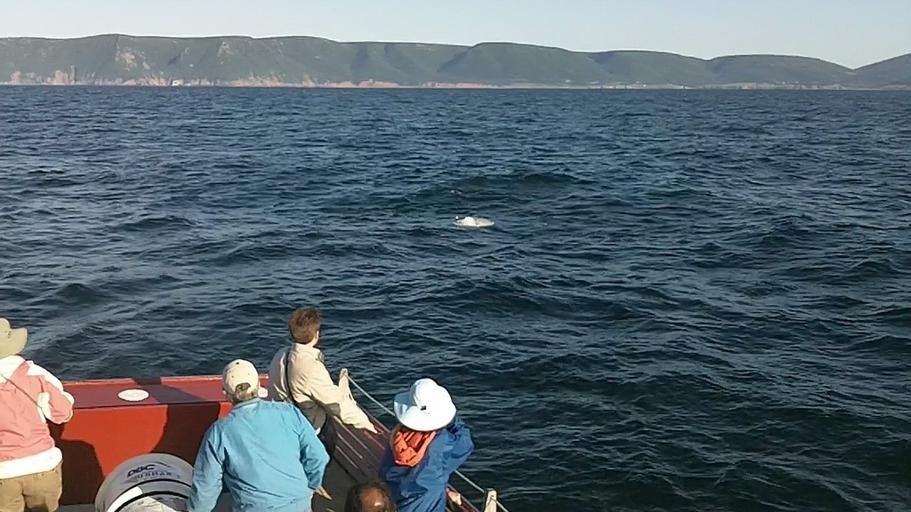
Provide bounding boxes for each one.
[316,414,337,461]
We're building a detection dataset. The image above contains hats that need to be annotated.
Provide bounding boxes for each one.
[0,317,26,360]
[392,377,456,432]
[221,359,259,397]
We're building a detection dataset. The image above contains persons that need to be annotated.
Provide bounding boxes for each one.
[379,378,475,512]
[343,482,397,512]
[183,358,331,512]
[267,306,378,512]
[0,317,74,512]
